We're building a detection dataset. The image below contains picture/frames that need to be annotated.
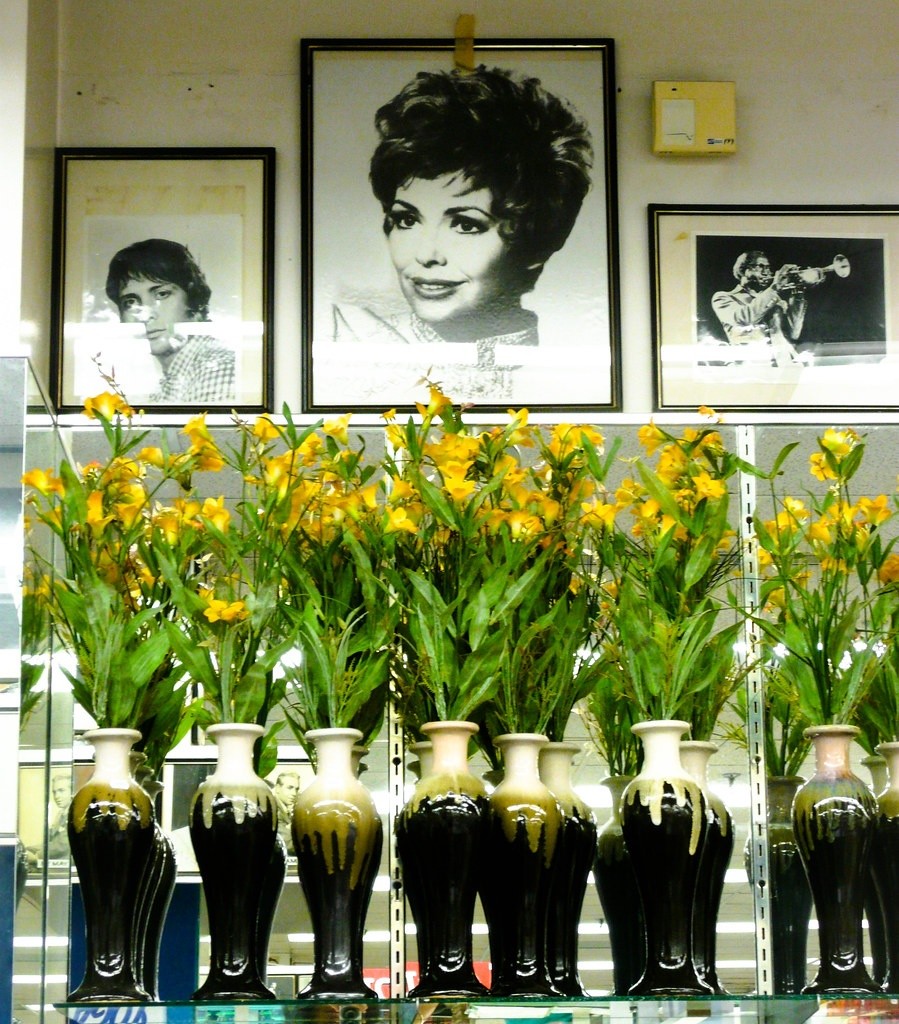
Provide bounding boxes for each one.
[644,203,899,414]
[49,145,277,415]
[295,32,630,416]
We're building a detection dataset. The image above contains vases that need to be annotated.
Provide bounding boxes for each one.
[57,717,899,1000]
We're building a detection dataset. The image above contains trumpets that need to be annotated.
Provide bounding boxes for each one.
[762,254,850,278]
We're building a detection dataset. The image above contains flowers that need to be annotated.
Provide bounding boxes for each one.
[18,374,897,740]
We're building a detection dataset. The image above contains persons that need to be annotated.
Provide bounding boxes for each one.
[328,60,595,398]
[712,249,808,383]
[104,239,236,403]
